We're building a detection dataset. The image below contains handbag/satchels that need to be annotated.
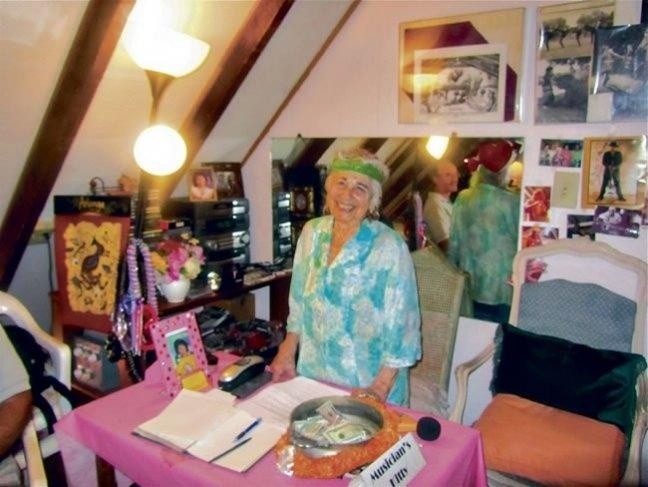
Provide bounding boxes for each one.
[4,325,50,385]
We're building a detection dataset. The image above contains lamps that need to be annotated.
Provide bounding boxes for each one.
[123,24,210,240]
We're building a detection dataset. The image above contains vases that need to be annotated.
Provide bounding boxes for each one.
[156,275,191,303]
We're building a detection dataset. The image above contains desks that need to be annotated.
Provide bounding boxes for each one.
[54,350,490,487]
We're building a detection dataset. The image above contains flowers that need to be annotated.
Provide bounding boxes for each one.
[151,233,206,285]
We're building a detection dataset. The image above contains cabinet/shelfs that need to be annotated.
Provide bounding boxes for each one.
[48,268,292,400]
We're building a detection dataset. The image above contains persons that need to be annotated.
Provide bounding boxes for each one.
[0,323,32,461]
[446,162,521,323]
[173,337,194,365]
[428,68,482,112]
[539,20,604,51]
[270,146,424,410]
[190,172,214,199]
[524,141,628,247]
[424,158,461,250]
[542,65,555,111]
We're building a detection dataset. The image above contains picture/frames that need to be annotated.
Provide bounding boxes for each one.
[150,310,212,399]
[533,0,619,123]
[582,135,646,209]
[396,8,525,122]
[187,166,218,202]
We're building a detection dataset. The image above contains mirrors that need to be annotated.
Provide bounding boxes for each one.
[270,136,523,326]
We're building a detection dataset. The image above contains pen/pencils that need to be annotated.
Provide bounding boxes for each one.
[233,417,263,443]
[208,438,252,464]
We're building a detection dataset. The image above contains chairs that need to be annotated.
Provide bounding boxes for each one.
[448,236,647,487]
[410,245,465,391]
[0,291,72,470]
[22,419,49,487]
[461,276,476,318]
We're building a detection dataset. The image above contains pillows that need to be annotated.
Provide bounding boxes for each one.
[490,320,648,435]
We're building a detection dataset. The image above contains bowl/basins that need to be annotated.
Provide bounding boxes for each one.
[229,319,286,364]
[289,394,385,459]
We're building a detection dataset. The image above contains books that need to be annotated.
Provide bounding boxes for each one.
[131,387,287,473]
